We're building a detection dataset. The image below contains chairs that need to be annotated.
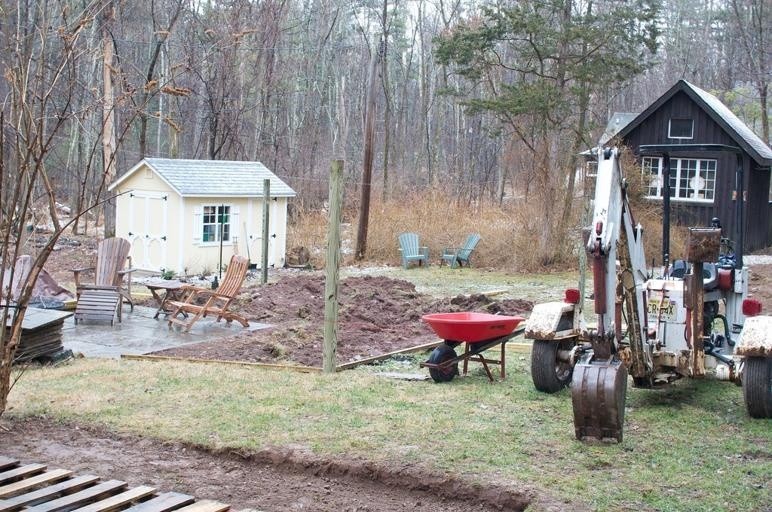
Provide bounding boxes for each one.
[68,237,137,326]
[397,230,481,270]
[164,254,249,330]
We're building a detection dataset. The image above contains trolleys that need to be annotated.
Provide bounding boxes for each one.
[419,311,525,383]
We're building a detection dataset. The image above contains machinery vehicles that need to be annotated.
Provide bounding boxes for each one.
[524,146,772,442]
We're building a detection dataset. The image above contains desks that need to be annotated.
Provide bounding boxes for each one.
[143,278,192,318]
